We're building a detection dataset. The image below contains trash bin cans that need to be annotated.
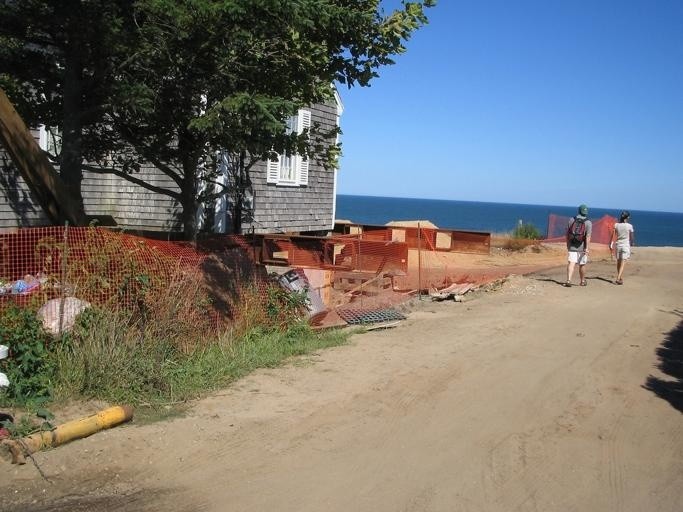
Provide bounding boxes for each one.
[277,268,328,324]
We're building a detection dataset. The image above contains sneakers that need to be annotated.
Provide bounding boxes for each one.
[581,280,587,286]
[566,280,570,286]
[616,278,624,284]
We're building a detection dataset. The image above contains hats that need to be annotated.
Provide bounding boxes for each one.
[579,205,588,215]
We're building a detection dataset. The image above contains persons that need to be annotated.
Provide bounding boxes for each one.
[608,211,633,284]
[565,205,592,287]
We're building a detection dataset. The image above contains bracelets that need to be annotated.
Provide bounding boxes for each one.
[609,241,614,249]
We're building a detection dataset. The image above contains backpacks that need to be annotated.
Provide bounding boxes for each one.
[568,216,589,248]
